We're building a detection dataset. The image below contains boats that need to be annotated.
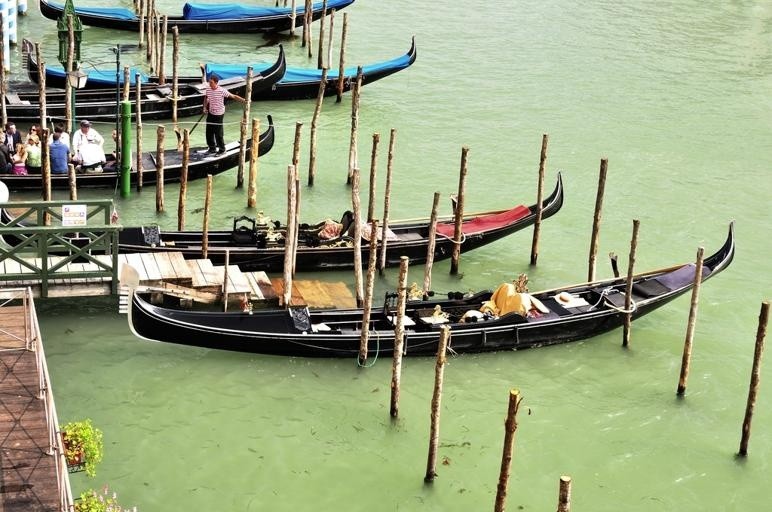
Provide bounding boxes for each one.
[109,168,565,276]
[38,0,357,36]
[0,113,277,195]
[20,34,417,103]
[0,44,286,124]
[119,218,737,362]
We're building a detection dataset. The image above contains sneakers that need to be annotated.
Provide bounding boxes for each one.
[205,149,225,156]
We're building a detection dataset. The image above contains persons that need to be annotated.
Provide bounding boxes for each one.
[202,76,251,157]
[0,119,134,174]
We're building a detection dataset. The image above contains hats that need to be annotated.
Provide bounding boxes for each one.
[80,120,92,127]
